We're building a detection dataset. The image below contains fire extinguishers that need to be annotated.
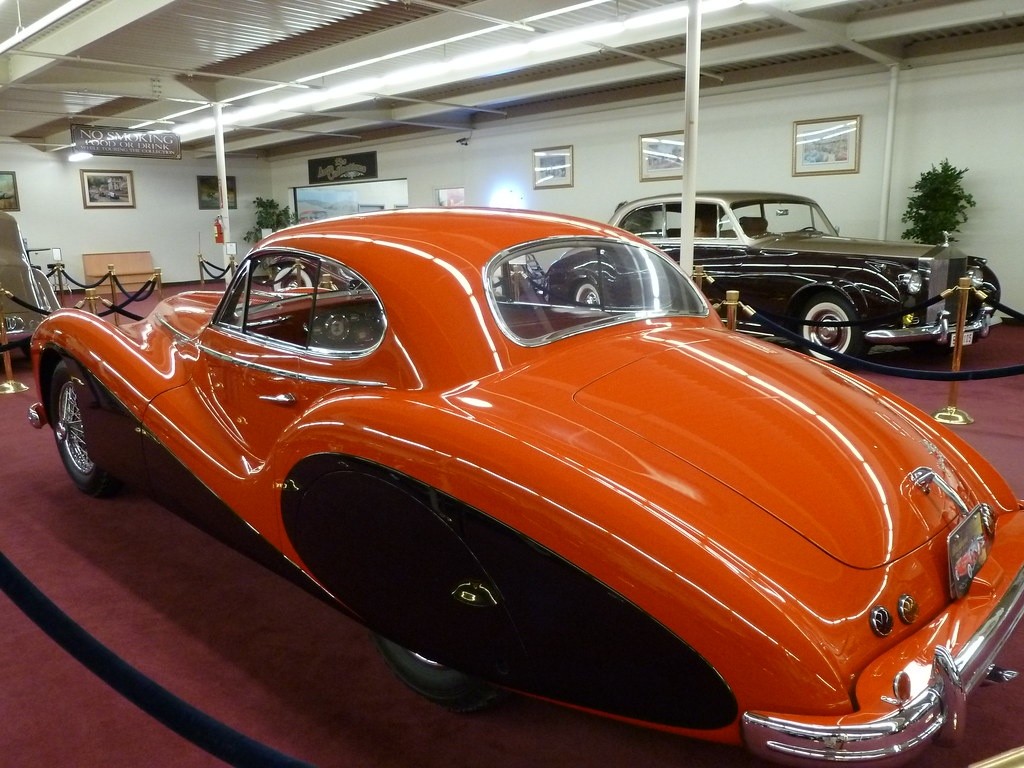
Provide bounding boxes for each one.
[214,215,224,243]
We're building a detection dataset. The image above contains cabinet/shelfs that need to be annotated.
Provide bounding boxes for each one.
[84,250,157,294]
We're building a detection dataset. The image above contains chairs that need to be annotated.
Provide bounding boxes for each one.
[739,216,768,238]
[695,217,717,236]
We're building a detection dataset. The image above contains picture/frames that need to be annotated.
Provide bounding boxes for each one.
[792,115,862,177]
[638,130,683,182]
[531,144,574,189]
[196,175,237,210]
[79,169,137,210]
[0,171,21,212]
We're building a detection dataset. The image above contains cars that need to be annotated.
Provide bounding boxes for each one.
[0,209,62,359]
[266,249,360,294]
[544,191,1001,366]
[29,208,1024,768]
